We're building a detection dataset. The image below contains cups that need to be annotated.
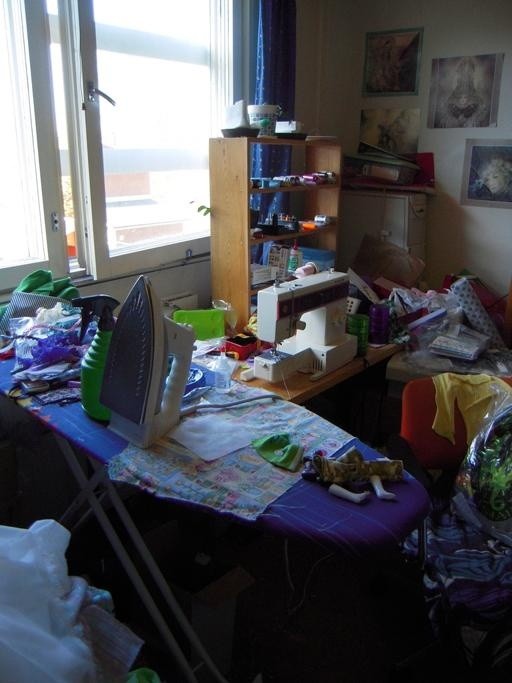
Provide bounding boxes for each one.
[346,314,369,357]
[369,303,390,344]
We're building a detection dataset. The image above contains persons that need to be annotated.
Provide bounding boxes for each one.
[473,155,512,204]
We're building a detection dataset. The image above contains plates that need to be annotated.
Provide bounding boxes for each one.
[306,135,337,141]
[274,133,307,140]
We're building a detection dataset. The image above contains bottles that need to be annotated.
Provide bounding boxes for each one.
[250,118,271,130]
[287,238,299,273]
[294,261,318,278]
[214,348,232,394]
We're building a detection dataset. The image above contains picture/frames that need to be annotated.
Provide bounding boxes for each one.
[361,26,424,97]
[460,137,512,209]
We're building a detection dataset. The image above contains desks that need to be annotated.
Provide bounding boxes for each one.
[0,324,430,682]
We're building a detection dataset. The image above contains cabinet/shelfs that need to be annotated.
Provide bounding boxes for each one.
[340,192,426,262]
[208,138,341,331]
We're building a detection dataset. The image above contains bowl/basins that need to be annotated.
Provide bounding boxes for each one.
[222,129,260,138]
